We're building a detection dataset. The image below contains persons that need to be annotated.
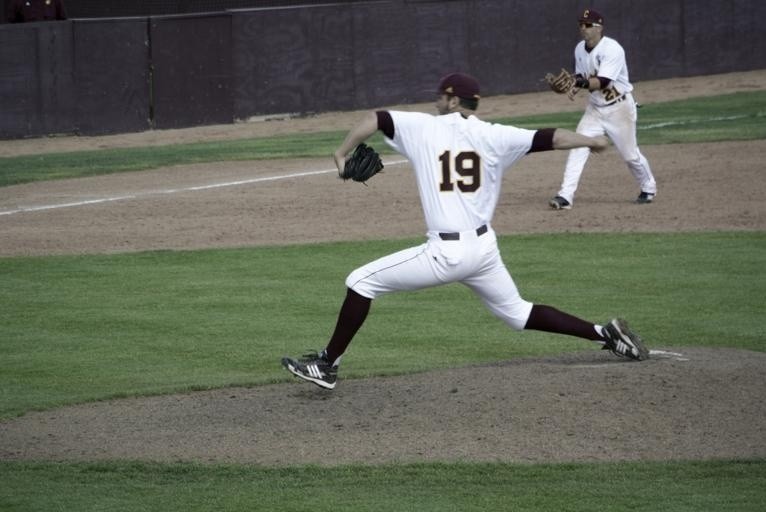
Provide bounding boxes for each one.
[545,10,657,209]
[281,74,651,390]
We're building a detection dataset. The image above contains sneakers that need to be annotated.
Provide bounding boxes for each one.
[281,348,339,389]
[549,196,572,210]
[637,192,654,203]
[601,316,650,361]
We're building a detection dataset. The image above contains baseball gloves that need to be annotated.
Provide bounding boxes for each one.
[340,143,383,182]
[547,69,577,93]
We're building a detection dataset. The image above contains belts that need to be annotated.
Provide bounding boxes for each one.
[440,224,486,241]
[605,95,625,105]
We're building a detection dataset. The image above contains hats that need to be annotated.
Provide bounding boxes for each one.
[423,72,481,101]
[577,9,604,25]
